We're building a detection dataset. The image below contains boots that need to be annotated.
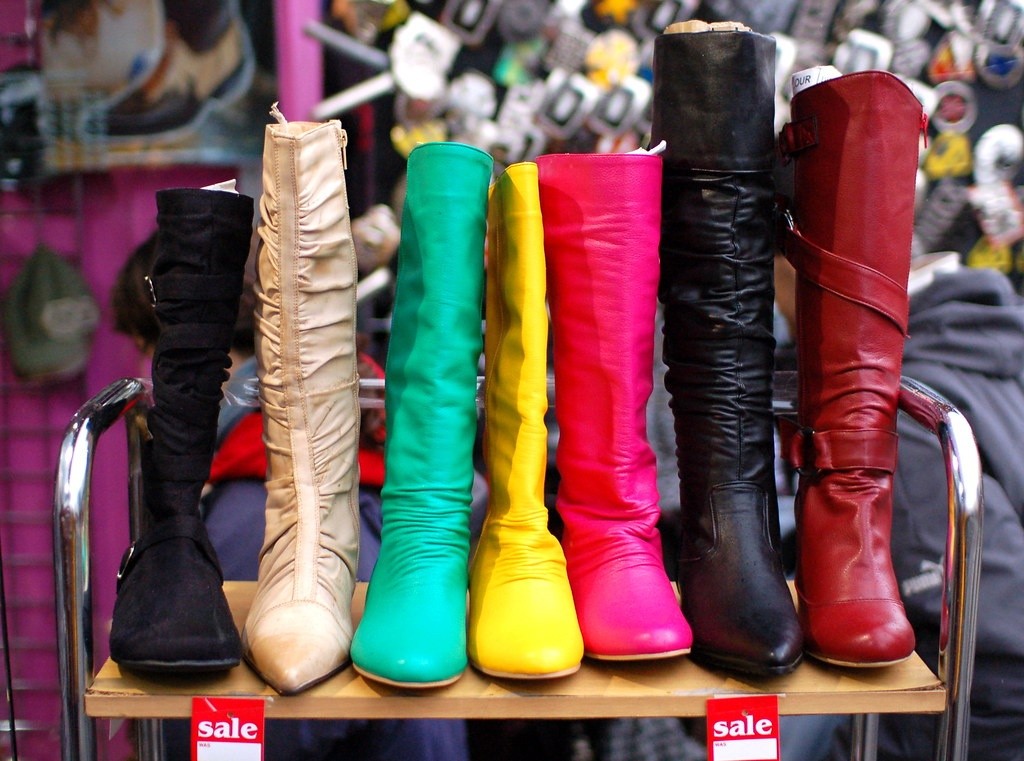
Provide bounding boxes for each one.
[781,70,915,669]
[351,142,495,689]
[537,150,693,662]
[108,186,253,680]
[651,31,800,679]
[82,0,256,143]
[245,102,362,693]
[469,162,583,680]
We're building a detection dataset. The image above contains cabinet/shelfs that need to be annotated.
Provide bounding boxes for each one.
[49,370,983,761]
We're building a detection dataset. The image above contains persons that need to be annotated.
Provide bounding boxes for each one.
[771,133,1024,761]
[108,226,467,761]
[524,300,845,761]
